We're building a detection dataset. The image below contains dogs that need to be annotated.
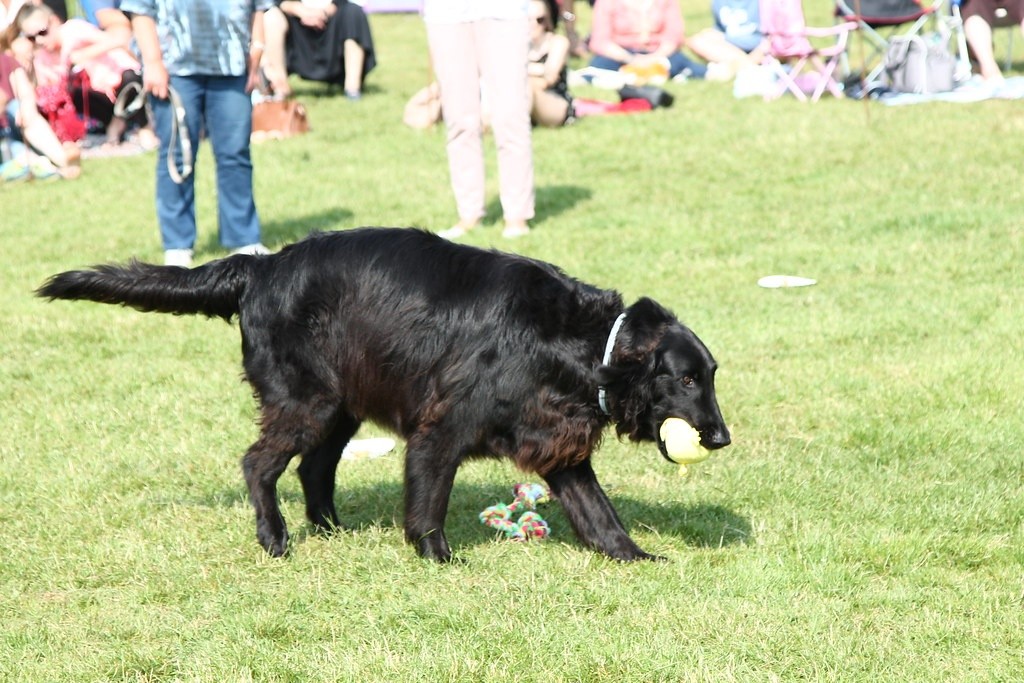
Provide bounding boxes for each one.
[30,224,730,565]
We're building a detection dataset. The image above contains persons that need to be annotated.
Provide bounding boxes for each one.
[0,0,1023,275]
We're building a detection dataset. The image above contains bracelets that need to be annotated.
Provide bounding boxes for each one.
[249,40,263,49]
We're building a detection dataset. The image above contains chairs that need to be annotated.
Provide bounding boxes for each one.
[832,0,963,98]
[754,0,858,105]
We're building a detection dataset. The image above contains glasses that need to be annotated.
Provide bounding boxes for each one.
[531,14,550,23]
[26,13,53,43]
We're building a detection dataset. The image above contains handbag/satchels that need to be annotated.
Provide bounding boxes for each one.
[887,31,957,93]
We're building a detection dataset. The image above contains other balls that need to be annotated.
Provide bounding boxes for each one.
[660,418,711,464]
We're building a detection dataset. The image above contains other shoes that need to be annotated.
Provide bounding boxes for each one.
[233,241,272,256]
[437,225,465,240]
[164,248,194,269]
[502,223,531,239]
